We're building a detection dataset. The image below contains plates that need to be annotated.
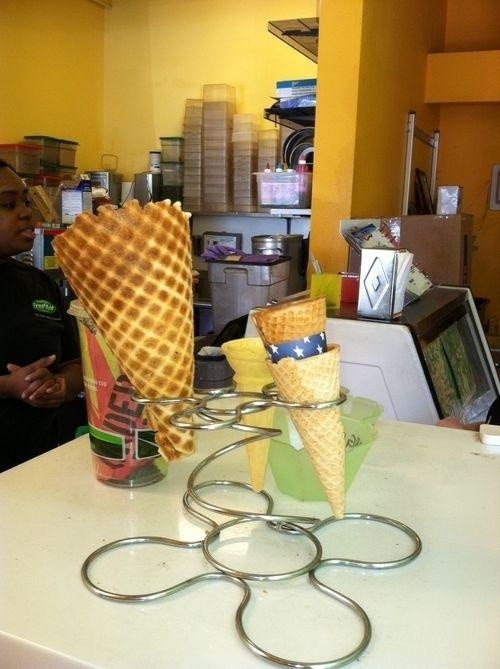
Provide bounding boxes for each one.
[480,421,500,445]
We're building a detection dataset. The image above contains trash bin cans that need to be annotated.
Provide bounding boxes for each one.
[206,254,291,333]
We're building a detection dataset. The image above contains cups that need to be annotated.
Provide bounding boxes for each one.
[64,296,172,488]
[337,272,360,303]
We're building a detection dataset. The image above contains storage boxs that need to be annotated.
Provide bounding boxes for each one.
[400,212,475,287]
[250,168,313,208]
[159,83,283,214]
[0,132,79,185]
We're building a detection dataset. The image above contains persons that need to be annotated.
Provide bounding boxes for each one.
[0,160,88,473]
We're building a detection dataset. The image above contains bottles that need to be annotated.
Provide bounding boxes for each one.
[149,150,161,172]
[260,157,309,205]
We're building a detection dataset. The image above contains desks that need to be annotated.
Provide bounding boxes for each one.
[1,394,499,666]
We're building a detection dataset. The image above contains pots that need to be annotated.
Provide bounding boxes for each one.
[250,233,304,275]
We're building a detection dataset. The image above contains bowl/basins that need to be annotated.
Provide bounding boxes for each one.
[266,430,382,504]
[270,389,384,448]
